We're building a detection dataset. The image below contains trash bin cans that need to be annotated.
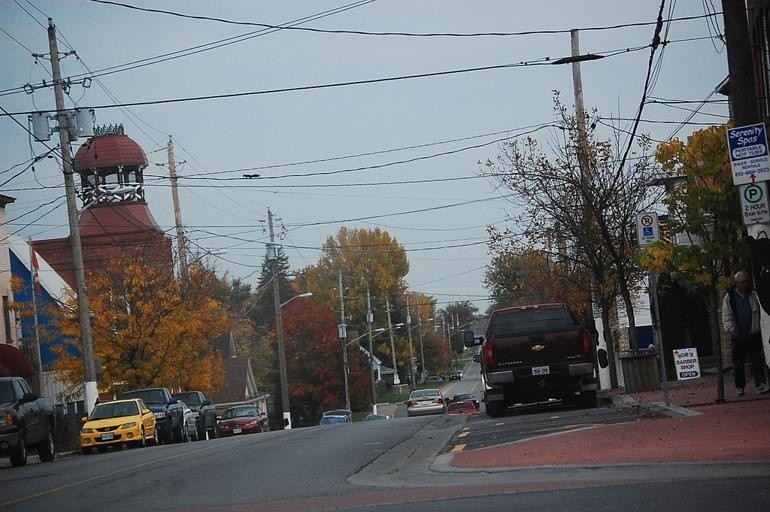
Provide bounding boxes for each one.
[619,348,662,394]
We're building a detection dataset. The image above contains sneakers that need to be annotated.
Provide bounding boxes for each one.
[736,387,744,395]
[756,383,770,395]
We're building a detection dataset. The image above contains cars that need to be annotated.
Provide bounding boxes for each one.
[317,408,391,425]
[402,388,481,416]
[79,388,272,454]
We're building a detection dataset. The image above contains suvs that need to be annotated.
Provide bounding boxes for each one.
[463,299,601,411]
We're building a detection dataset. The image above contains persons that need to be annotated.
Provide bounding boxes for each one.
[721,269,770,396]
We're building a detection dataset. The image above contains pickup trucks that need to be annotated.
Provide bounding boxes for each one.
[0,374,60,467]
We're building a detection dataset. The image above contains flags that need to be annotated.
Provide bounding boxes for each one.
[30,242,41,295]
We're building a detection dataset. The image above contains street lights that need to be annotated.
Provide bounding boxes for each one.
[338,317,474,407]
[273,288,318,434]
